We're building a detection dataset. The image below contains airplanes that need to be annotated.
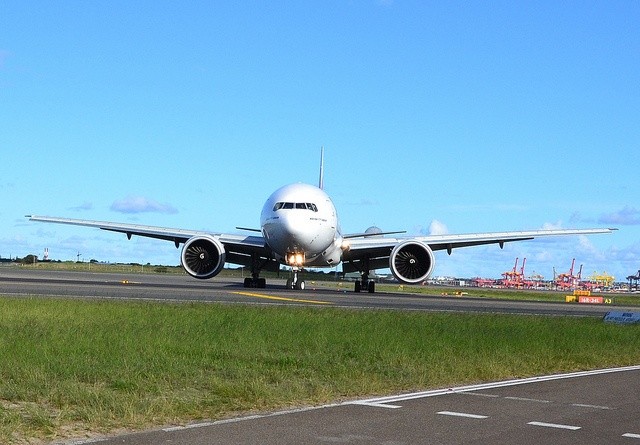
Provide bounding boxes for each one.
[23,144,620,293]
